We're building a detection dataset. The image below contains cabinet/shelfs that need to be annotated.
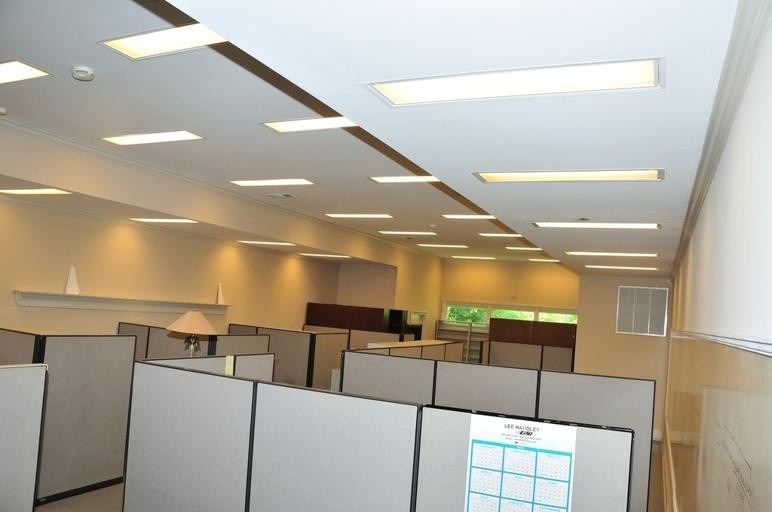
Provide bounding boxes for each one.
[435,318,489,364]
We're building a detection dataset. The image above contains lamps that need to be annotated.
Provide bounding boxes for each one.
[165,309,220,358]
